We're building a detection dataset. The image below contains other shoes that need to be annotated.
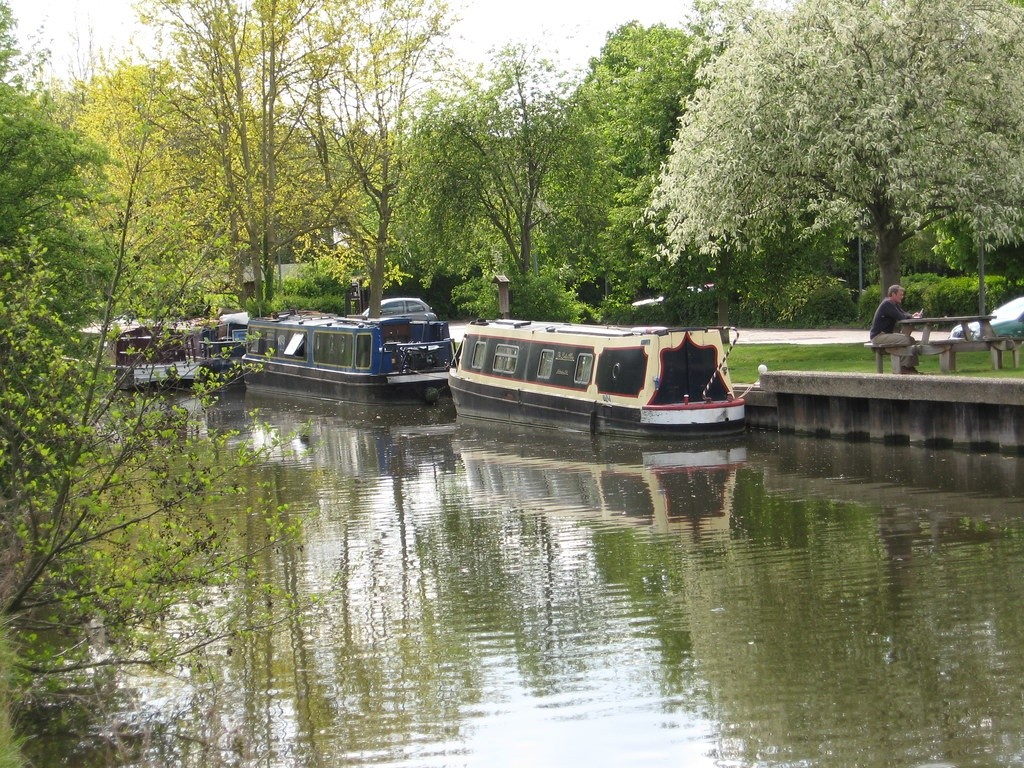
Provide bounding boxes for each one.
[909,366,923,374]
[901,366,914,374]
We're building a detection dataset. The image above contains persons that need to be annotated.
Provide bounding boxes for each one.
[870,285,923,374]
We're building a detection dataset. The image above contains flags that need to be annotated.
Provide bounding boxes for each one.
[333,231,350,249]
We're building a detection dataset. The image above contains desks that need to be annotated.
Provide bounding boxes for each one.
[899,316,998,341]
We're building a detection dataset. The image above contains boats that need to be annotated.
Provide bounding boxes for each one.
[240,308,458,405]
[446,315,748,437]
[60,311,248,391]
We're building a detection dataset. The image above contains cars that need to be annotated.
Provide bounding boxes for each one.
[361,297,439,321]
[948,293,1024,338]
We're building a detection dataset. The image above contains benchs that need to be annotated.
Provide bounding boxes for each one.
[864,339,1024,374]
